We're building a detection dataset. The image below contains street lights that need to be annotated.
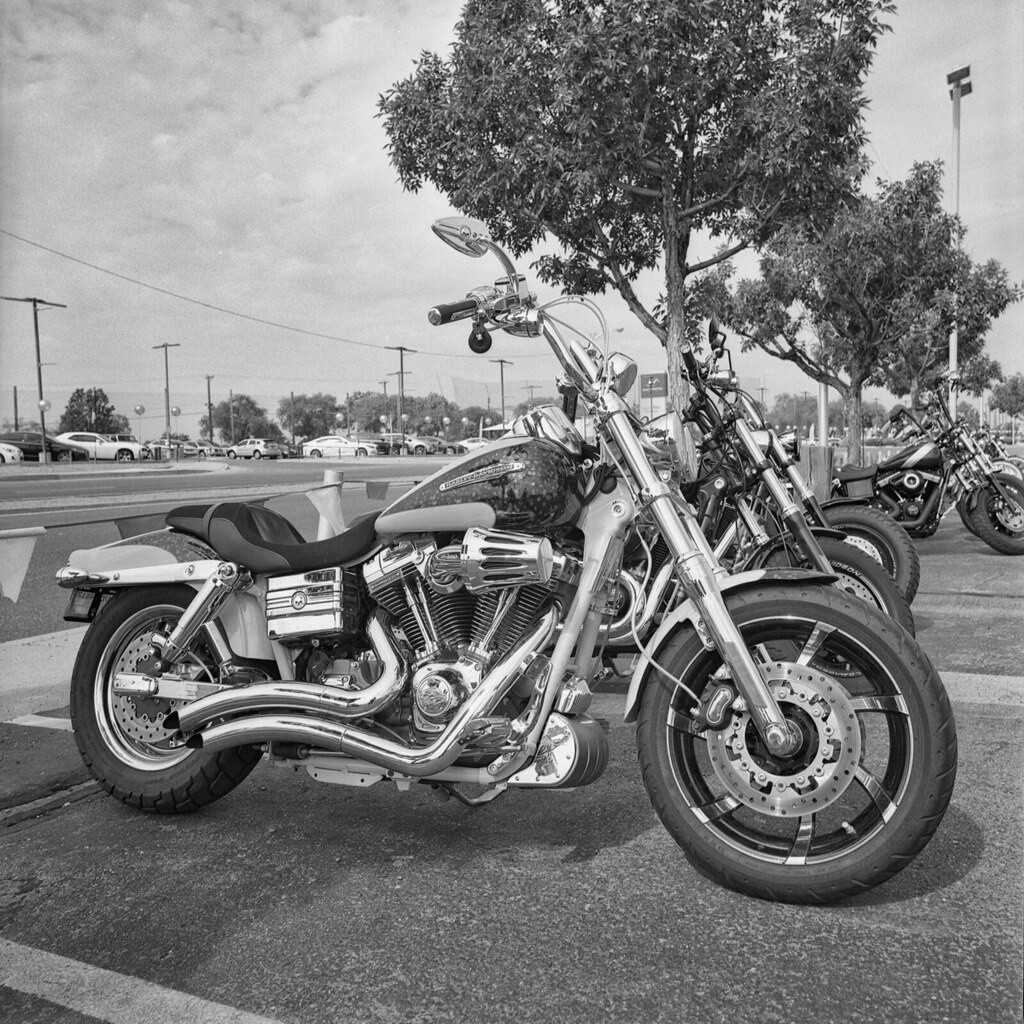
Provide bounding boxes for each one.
[947,65,974,424]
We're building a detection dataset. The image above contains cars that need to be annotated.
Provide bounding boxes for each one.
[276,432,495,459]
[226,437,280,460]
[302,436,379,459]
[0,431,90,463]
[0,442,24,465]
[55,431,149,464]
[105,428,223,463]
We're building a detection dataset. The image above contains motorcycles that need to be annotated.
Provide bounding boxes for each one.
[55,216,958,908]
[518,312,1024,696]
